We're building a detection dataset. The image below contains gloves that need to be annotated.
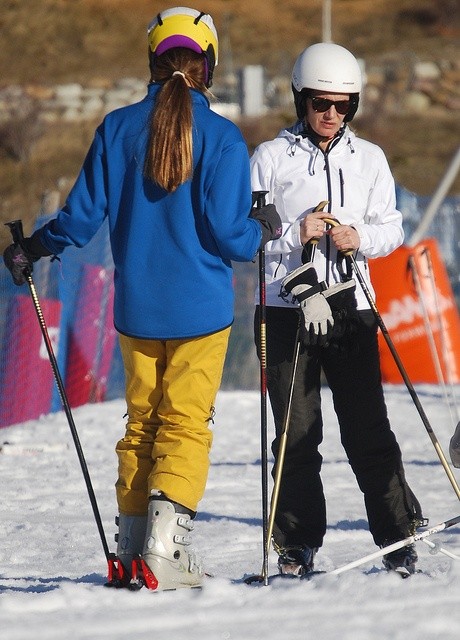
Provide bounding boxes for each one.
[320,279,366,354]
[279,261,335,348]
[249,203,282,248]
[3,231,51,286]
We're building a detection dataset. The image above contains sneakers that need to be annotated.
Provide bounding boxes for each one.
[383,540,419,577]
[276,546,317,578]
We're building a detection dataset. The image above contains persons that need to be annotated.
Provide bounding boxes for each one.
[251,42,423,578]
[4,6,283,591]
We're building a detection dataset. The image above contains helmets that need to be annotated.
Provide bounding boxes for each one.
[146,7,218,89]
[292,43,362,121]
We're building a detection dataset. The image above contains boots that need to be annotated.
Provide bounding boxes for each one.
[144,500,206,589]
[116,514,146,577]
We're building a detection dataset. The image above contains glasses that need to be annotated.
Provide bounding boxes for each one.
[308,95,353,114]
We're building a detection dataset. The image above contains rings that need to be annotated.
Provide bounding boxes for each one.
[315,226,319,231]
